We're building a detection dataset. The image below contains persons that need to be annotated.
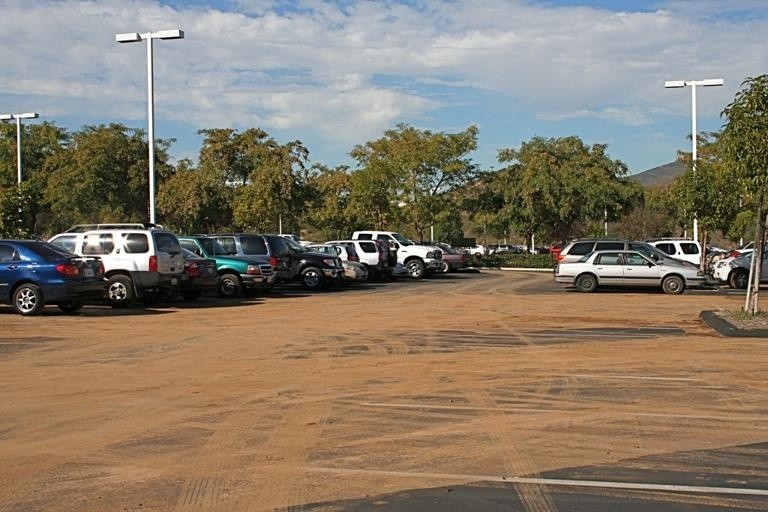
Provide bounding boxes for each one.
[377,239,391,283]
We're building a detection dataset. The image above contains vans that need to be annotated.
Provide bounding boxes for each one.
[641,237,703,268]
[556,237,700,269]
[192,233,294,292]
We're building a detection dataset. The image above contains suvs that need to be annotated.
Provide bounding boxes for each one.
[45,222,190,309]
[174,237,277,299]
[352,231,443,279]
[712,245,767,289]
[267,236,346,290]
[323,240,398,280]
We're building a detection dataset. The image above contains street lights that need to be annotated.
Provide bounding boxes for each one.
[115,29,185,231]
[664,78,725,241]
[1,113,39,240]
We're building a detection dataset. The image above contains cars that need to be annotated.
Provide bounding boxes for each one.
[461,244,489,256]
[704,236,767,275]
[488,242,562,255]
[301,246,368,287]
[553,250,706,295]
[0,239,107,316]
[180,246,219,300]
[277,234,316,247]
[708,251,754,278]
[303,243,360,261]
[413,242,473,274]
